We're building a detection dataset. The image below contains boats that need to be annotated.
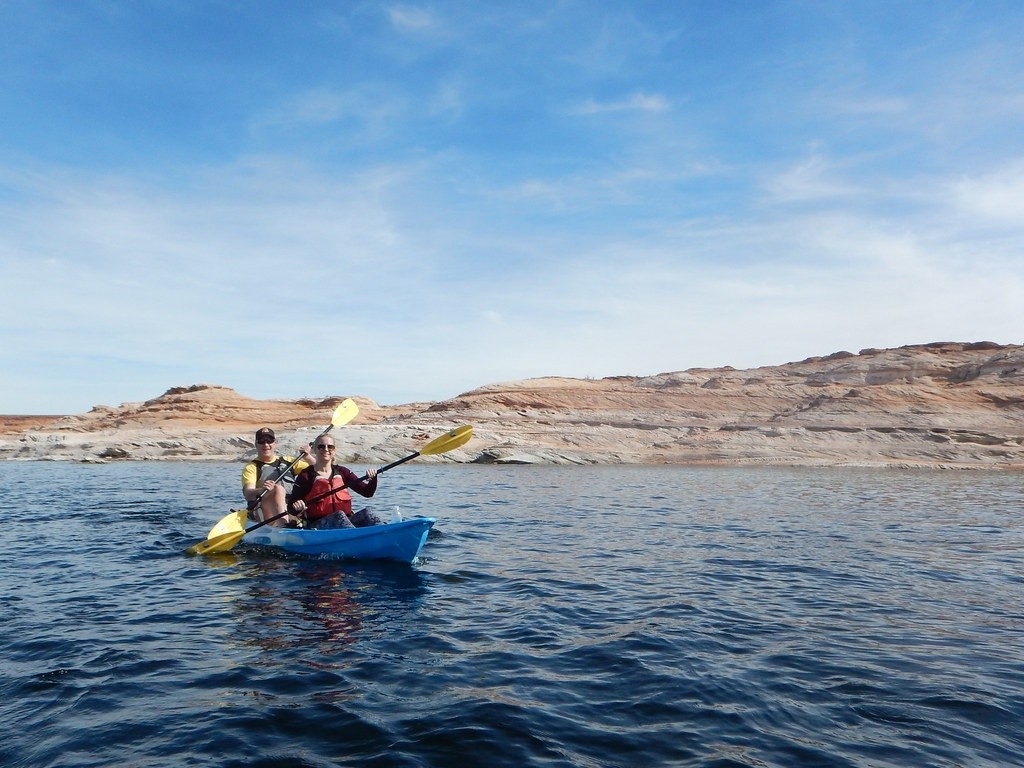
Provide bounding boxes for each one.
[240,518,436,567]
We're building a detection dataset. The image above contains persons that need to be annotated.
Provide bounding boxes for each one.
[242,427,317,528]
[287,433,381,530]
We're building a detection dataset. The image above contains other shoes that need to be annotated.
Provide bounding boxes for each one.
[286,520,296,528]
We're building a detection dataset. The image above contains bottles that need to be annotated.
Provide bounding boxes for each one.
[391,506,402,523]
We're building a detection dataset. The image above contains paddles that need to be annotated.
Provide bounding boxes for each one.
[186,424,475,555]
[208,398,362,539]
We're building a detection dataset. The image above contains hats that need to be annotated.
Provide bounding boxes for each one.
[256,427,275,439]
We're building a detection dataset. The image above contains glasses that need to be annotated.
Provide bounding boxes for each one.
[316,444,335,451]
[257,437,274,444]
